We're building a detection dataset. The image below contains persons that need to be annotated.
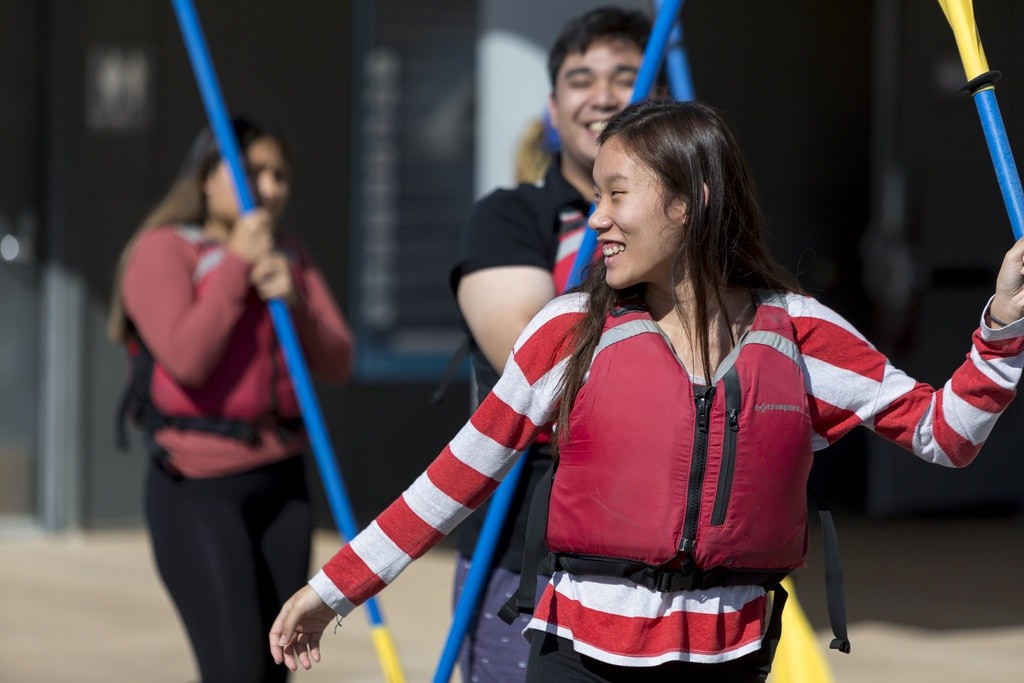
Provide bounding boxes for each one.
[269,97,1024,683]
[101,113,354,683]
[516,122,551,185]
[430,6,693,683]
